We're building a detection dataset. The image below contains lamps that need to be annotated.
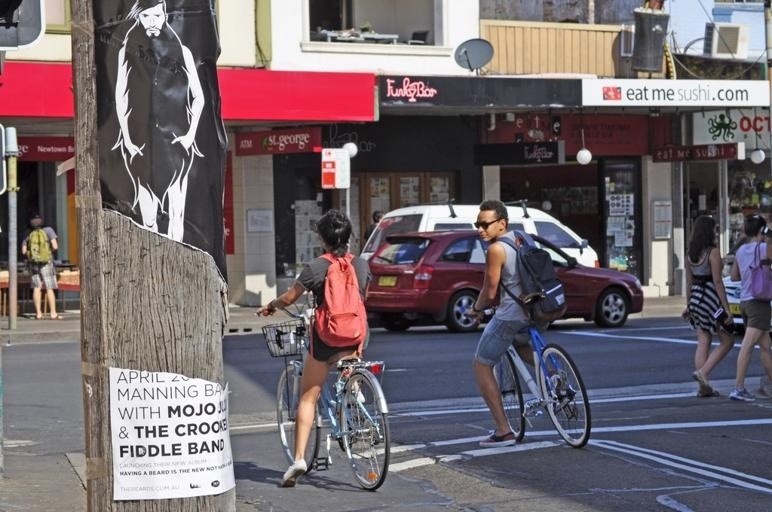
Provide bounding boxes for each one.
[748,106,765,165]
[343,120,359,157]
[573,109,593,166]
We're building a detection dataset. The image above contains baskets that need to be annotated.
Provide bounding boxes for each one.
[261,320,308,358]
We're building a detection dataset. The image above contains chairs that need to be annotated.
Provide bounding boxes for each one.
[402,29,431,44]
[0,258,81,323]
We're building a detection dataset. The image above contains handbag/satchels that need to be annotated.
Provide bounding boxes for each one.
[714,309,736,334]
[749,264,772,300]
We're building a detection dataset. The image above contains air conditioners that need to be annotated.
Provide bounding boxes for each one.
[701,19,750,61]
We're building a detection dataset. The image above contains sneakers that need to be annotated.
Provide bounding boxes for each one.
[556,386,575,412]
[479,431,517,448]
[693,371,713,393]
[348,390,366,406]
[729,388,756,402]
[697,390,720,397]
[281,459,307,487]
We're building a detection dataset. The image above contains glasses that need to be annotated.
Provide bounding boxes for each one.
[474,219,500,229]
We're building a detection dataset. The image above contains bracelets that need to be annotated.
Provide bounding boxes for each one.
[471,303,481,313]
[269,300,275,309]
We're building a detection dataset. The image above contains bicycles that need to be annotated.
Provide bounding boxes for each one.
[254,294,390,491]
[463,303,591,448]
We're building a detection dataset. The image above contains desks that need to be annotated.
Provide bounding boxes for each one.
[320,28,399,45]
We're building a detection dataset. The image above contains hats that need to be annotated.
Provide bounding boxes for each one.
[30,218,41,227]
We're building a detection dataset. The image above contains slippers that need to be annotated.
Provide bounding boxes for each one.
[50,314,64,320]
[35,317,45,320]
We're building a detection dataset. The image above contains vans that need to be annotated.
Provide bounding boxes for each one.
[359,198,600,328]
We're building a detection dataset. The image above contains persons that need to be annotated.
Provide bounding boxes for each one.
[22,211,63,320]
[681,216,735,397]
[729,213,772,401]
[467,200,574,446]
[110,0,204,242]
[261,208,369,487]
[364,211,383,239]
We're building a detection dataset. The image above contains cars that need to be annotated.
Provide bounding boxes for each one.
[364,229,644,332]
[721,227,772,340]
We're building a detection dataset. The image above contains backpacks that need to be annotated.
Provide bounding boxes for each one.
[315,254,368,347]
[494,229,567,320]
[27,229,49,263]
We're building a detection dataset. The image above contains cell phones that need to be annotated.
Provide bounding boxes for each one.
[760,226,772,237]
